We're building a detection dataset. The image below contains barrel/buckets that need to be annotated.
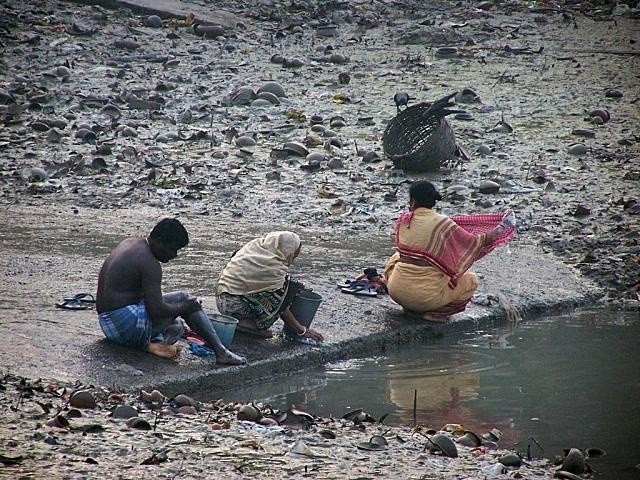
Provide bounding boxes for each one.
[205,313,239,348]
[283,288,324,339]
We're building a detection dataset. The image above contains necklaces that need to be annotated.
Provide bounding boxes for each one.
[144,237,151,244]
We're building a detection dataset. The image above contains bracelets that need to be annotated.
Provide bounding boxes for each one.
[296,325,307,337]
[176,303,184,317]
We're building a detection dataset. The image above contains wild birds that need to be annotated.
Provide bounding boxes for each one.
[393,91,417,112]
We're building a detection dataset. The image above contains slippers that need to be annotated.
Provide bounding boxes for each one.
[337,279,378,297]
[235,321,272,339]
[55,292,96,310]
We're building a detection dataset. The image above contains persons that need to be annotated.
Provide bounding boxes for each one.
[213,230,326,345]
[383,179,516,327]
[96,217,249,367]
